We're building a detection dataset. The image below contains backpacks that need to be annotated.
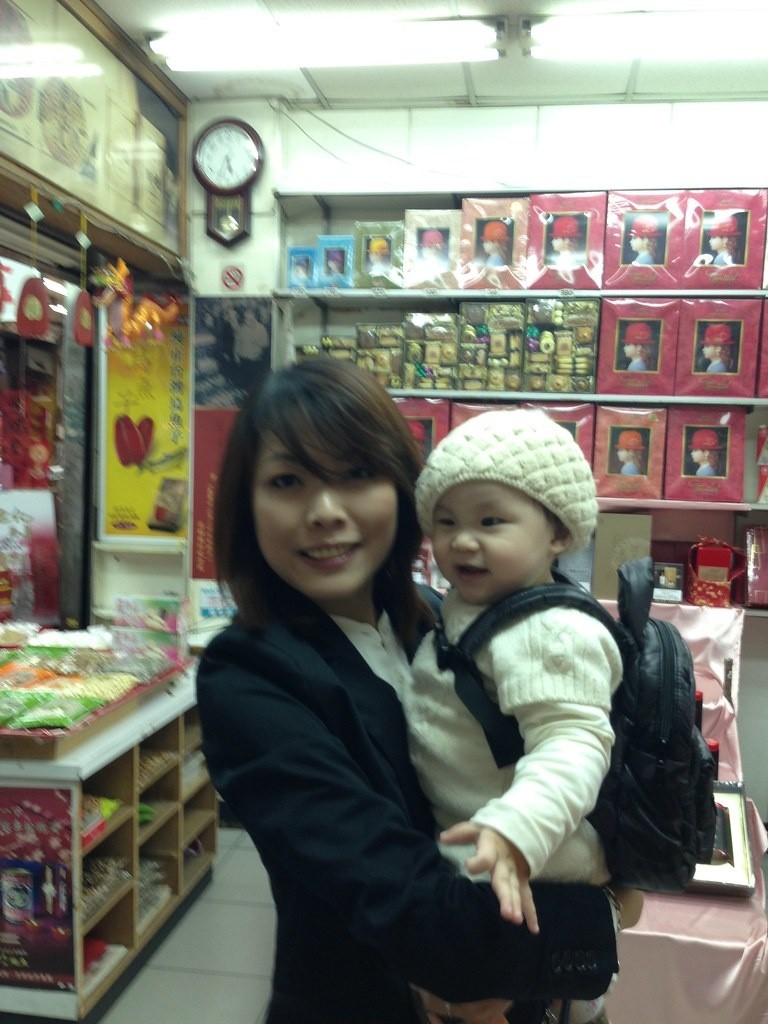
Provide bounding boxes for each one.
[445,554,718,898]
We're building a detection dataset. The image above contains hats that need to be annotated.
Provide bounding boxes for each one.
[412,407,599,559]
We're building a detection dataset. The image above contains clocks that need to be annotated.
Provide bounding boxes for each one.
[191,116,265,249]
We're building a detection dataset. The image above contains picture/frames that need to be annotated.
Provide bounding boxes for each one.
[682,781,757,898]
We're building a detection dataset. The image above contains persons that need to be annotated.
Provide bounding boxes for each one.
[408,411,622,1024]
[198,357,645,1024]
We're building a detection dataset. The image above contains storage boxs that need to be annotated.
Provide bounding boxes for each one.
[523,297,600,394]
[461,197,530,289]
[593,405,668,500]
[457,302,526,393]
[681,190,768,289]
[313,234,354,289]
[596,298,681,395]
[294,312,459,390]
[450,401,516,433]
[402,209,461,289]
[602,191,682,289]
[287,245,314,290]
[391,398,450,472]
[526,191,608,291]
[352,220,403,290]
[673,298,762,398]
[663,405,746,503]
[519,401,596,472]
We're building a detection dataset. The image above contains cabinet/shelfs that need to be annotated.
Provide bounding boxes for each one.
[0,648,219,1023]
[275,288,768,619]
